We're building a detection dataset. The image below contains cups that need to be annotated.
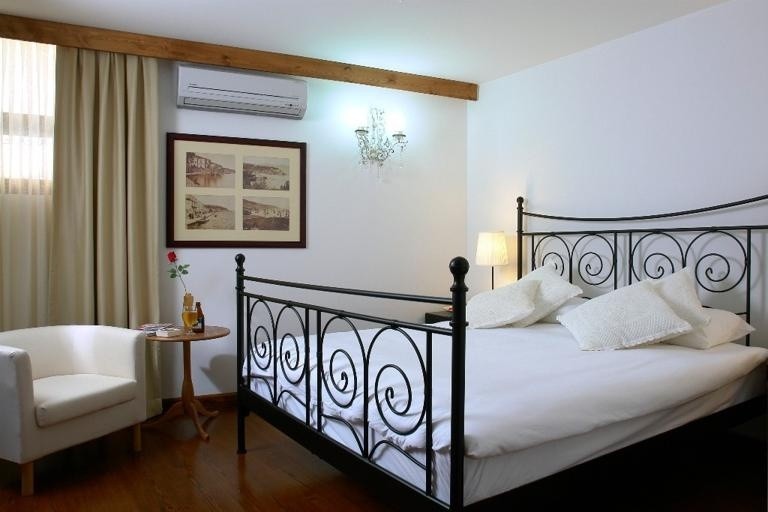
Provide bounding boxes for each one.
[182,305,198,335]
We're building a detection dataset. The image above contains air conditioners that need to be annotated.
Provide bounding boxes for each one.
[175,63,308,121]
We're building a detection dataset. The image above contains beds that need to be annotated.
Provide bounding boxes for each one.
[235,196,767,512]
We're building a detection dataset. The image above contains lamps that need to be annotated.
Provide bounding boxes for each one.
[474,231,509,290]
[355,125,408,168]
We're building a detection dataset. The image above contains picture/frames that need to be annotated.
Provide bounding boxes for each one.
[165,131,307,249]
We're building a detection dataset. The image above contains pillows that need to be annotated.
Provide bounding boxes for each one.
[539,297,590,324]
[661,306,756,349]
[508,262,583,327]
[466,279,541,330]
[556,280,693,352]
[653,267,711,329]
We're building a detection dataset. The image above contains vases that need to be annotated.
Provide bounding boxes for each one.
[181,293,193,334]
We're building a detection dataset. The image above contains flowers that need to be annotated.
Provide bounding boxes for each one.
[166,251,190,293]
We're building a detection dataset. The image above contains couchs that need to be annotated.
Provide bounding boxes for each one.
[0,323,147,495]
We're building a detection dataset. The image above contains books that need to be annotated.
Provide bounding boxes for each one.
[135,322,174,337]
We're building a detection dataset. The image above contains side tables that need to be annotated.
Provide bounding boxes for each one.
[139,324,231,442]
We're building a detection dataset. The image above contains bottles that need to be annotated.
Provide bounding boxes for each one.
[192,302,204,333]
